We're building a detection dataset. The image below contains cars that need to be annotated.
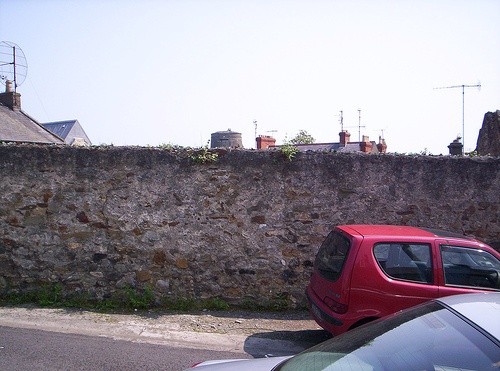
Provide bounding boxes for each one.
[185,292,500,371]
[303,225,500,337]
